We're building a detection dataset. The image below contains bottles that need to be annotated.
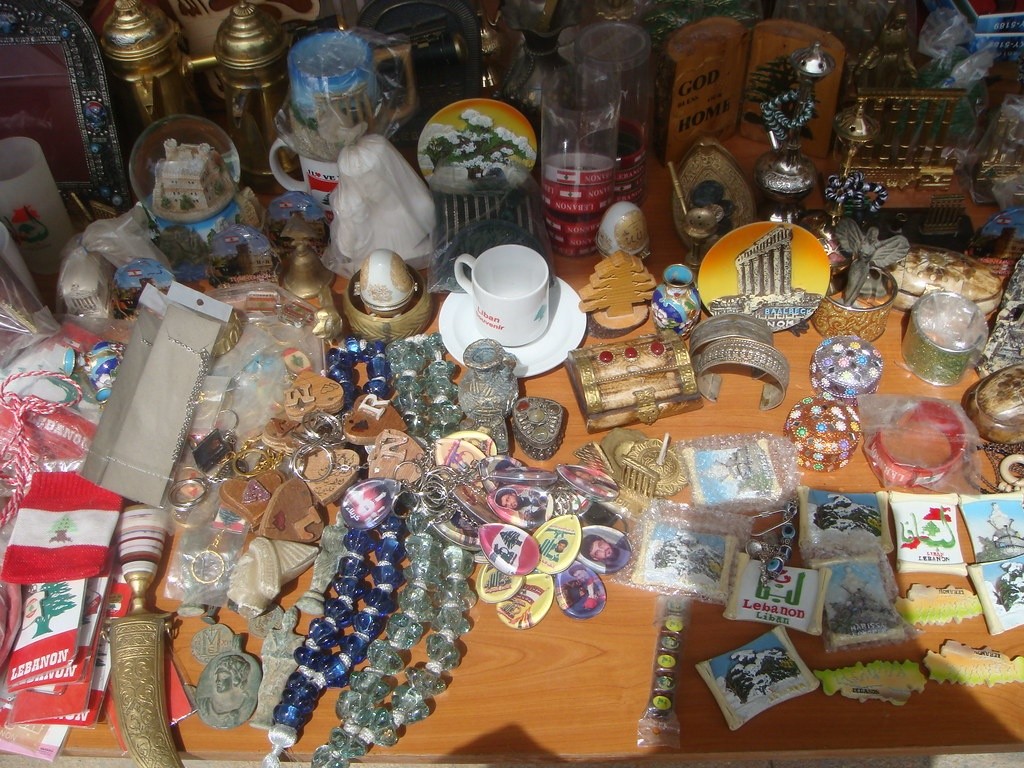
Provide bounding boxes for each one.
[491,0,581,141]
[651,263,701,340]
[573,22,652,206]
[541,64,622,257]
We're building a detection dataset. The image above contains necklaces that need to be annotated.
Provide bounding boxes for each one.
[261,332,475,768]
[743,499,804,577]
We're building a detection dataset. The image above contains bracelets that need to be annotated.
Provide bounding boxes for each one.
[866,396,969,489]
[689,313,791,411]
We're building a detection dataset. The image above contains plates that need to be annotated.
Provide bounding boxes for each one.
[438,274,587,378]
[417,99,538,188]
[697,224,830,332]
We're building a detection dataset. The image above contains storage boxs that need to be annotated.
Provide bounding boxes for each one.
[924,0,1024,65]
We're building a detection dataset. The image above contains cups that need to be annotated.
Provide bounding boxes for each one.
[288,31,419,147]
[0,136,74,274]
[453,244,550,347]
[269,135,338,227]
